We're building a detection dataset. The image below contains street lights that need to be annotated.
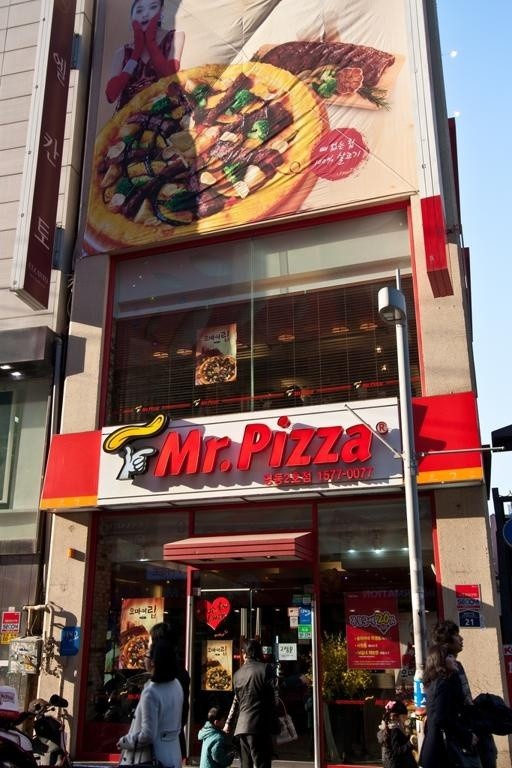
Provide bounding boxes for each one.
[377,268,431,758]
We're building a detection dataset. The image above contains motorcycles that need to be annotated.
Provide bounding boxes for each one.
[98,668,142,725]
[20,690,74,767]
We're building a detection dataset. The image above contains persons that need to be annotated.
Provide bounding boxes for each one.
[419,644,484,768]
[432,619,497,768]
[105,0,185,108]
[117,642,184,767]
[224,639,283,768]
[149,622,190,766]
[378,701,416,767]
[196,707,236,768]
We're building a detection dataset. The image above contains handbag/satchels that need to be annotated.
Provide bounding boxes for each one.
[276,714,298,744]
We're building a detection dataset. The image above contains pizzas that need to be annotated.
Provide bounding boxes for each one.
[196,353,239,387]
[205,666,233,691]
[87,62,325,247]
[121,634,151,669]
